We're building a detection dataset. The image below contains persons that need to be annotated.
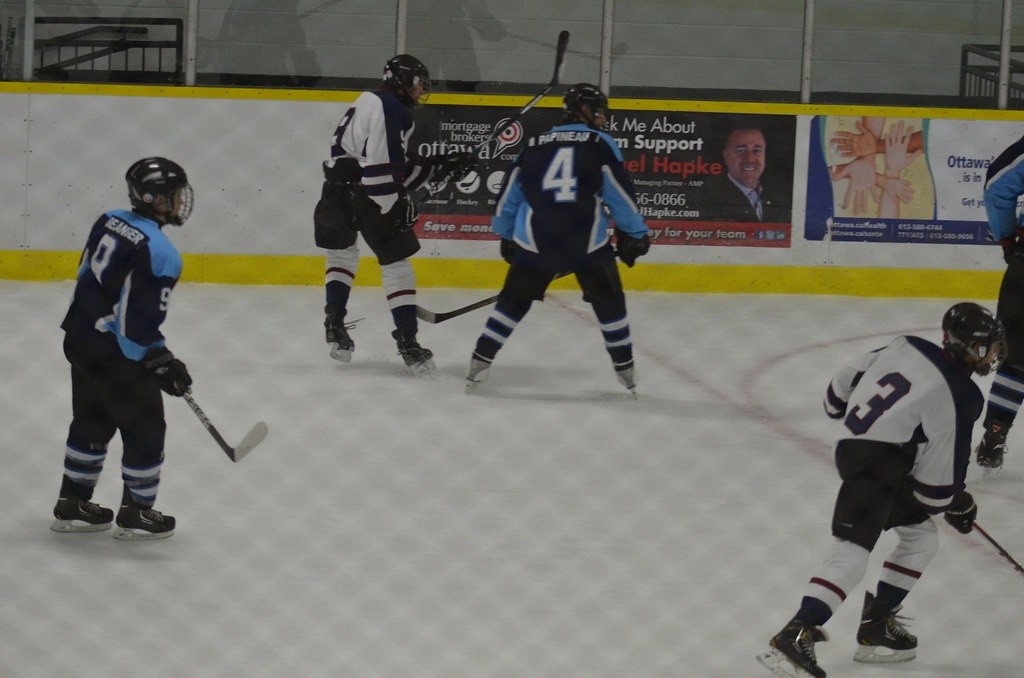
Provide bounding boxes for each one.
[49,156,192,540]
[464,83,651,399]
[825,114,926,219]
[314,54,436,375]
[682,123,790,224]
[757,303,1010,678]
[974,133,1024,483]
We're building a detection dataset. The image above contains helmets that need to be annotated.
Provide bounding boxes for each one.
[564,82,608,133]
[941,301,1007,376]
[380,55,431,111]
[124,156,194,227]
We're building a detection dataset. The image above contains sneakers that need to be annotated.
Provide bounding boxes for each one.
[617,362,639,397]
[323,301,356,363]
[757,617,832,677]
[465,350,492,391]
[973,419,1011,481]
[852,590,920,663]
[390,325,436,375]
[112,494,178,540]
[51,475,116,532]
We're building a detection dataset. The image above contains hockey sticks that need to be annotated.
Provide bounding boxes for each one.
[417,30,569,201]
[970,521,1024,573]
[185,391,268,462]
[417,252,620,324]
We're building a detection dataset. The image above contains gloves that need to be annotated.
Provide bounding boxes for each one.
[394,193,420,233]
[611,229,650,267]
[435,153,473,185]
[498,237,518,265]
[997,231,1024,273]
[150,348,193,396]
[943,491,979,534]
